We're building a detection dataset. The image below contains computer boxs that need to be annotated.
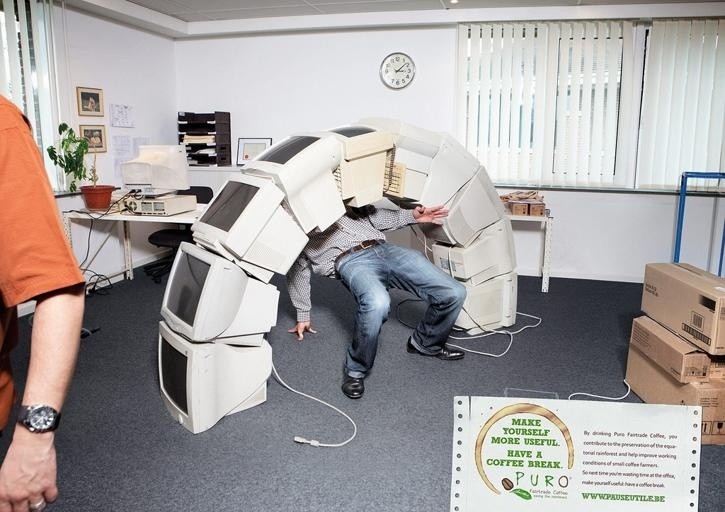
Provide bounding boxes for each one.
[120,195,197,216]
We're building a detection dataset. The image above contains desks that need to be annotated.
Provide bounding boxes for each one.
[509,213,553,293]
[62,203,212,280]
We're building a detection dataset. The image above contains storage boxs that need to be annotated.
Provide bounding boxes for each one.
[625,261,724,447]
[509,200,545,216]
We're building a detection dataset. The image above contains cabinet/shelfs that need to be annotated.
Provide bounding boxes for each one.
[177,112,231,167]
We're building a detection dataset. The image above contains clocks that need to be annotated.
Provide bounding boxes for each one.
[379,52,415,90]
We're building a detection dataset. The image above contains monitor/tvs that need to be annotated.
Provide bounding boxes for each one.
[119,144,189,198]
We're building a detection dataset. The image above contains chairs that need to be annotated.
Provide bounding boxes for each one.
[143,186,213,284]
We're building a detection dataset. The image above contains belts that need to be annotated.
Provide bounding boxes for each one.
[335,239,385,263]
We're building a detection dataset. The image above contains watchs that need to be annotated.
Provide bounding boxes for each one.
[14,405,62,434]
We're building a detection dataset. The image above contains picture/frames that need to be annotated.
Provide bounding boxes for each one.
[237,138,273,166]
[76,87,108,153]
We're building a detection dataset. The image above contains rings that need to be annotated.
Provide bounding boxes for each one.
[30,499,46,512]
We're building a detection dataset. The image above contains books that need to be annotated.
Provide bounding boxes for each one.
[184,134,216,154]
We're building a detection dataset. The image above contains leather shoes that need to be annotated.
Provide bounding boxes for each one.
[407,336,464,360]
[342,376,364,397]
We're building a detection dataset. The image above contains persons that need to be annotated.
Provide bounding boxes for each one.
[1,91,91,512]
[283,204,467,398]
[89,96,96,112]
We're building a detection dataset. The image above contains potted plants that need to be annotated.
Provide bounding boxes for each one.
[46,122,115,212]
